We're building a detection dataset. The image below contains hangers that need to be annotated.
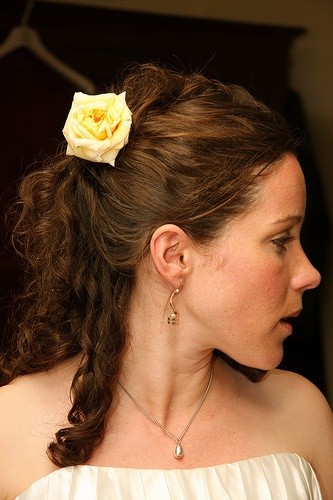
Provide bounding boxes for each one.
[0,0,98,96]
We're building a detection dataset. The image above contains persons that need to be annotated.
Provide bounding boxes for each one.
[0,64,333,500]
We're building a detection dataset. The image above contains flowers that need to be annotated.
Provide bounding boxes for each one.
[62,90,133,167]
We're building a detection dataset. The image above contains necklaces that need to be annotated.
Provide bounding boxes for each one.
[117,366,213,459]
